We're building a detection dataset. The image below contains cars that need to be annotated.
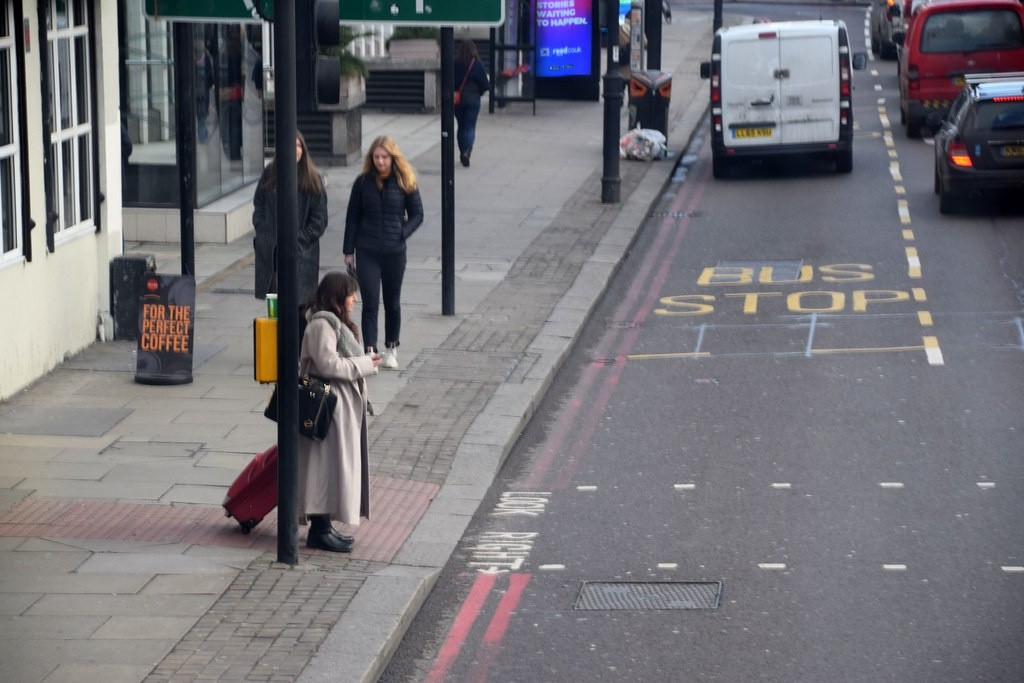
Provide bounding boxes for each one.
[927,73,1024,214]
[868,0,903,60]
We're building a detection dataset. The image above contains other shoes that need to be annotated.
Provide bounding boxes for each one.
[460,150,469,167]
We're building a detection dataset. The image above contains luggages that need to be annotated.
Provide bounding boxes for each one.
[221,443,278,534]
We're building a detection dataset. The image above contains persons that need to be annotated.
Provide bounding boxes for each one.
[299,269,383,552]
[455,38,489,166]
[191,24,264,161]
[121,122,132,202]
[947,10,1010,52]
[342,136,424,375]
[253,131,328,310]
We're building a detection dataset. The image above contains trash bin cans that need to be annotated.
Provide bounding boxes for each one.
[112,253,156,340]
[628,69,672,160]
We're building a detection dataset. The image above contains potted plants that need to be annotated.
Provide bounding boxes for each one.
[319,24,446,113]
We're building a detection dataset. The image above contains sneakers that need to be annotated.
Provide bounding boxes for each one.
[383,349,398,368]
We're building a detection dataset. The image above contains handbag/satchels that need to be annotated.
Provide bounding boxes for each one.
[454,91,460,104]
[265,377,340,441]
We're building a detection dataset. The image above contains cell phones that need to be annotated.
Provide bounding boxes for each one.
[379,351,387,359]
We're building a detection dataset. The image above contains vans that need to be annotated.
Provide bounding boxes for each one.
[698,18,870,178]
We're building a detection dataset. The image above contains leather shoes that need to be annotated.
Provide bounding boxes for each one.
[329,525,355,544]
[308,529,353,551]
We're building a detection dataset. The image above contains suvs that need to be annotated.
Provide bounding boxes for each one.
[891,0,1024,138]
